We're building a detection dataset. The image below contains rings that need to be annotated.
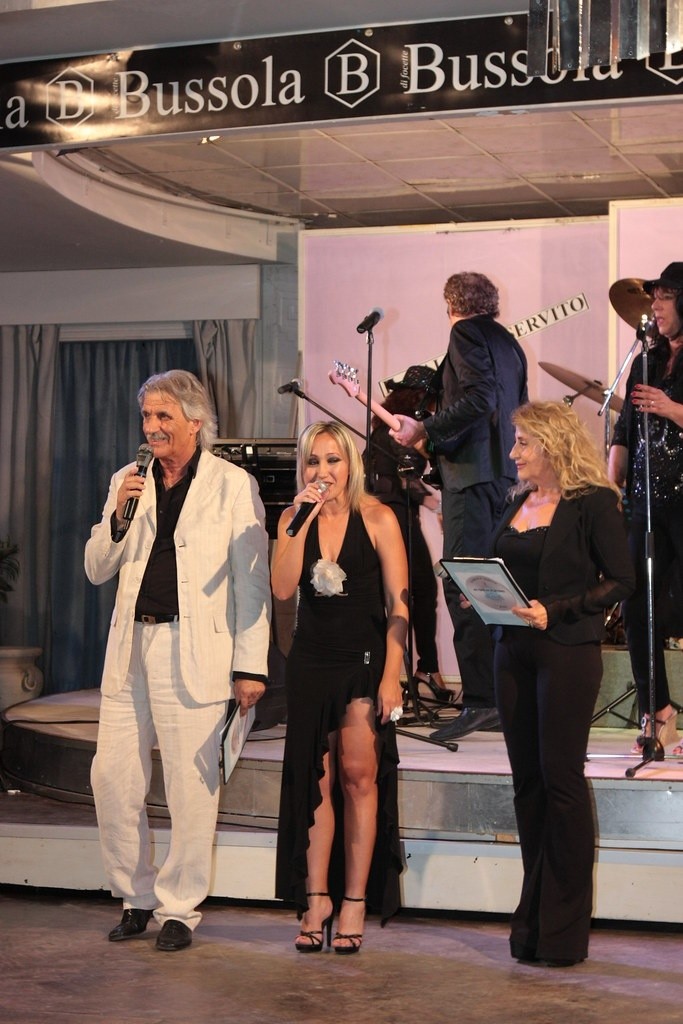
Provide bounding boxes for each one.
[391,708,403,721]
[651,400,655,404]
[529,620,533,626]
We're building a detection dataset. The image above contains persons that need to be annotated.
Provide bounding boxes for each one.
[362,365,451,701]
[389,272,528,740]
[606,262,683,756]
[271,421,407,949]
[84,369,273,951]
[460,400,636,967]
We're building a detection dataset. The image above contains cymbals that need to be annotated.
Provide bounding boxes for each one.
[608,277,655,338]
[538,360,625,414]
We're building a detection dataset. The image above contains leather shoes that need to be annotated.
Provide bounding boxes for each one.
[429,706,504,741]
[110,908,155,941]
[156,920,193,951]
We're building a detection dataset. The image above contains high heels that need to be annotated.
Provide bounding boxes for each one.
[296,892,335,952]
[631,707,678,755]
[332,897,367,955]
[413,668,455,702]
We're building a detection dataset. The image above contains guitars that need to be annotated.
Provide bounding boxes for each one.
[328,359,433,461]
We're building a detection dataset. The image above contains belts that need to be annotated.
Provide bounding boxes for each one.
[133,612,178,625]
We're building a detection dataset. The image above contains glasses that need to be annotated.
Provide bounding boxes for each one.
[653,290,681,300]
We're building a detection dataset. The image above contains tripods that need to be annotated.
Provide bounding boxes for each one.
[294,391,464,720]
[582,335,683,778]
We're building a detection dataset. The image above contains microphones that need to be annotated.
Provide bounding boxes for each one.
[357,307,385,334]
[277,378,303,394]
[124,444,154,520]
[286,481,328,537]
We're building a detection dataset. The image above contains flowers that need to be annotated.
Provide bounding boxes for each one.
[309,558,349,597]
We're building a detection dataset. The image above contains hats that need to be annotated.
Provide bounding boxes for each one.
[644,262,683,295]
[387,365,441,399]
